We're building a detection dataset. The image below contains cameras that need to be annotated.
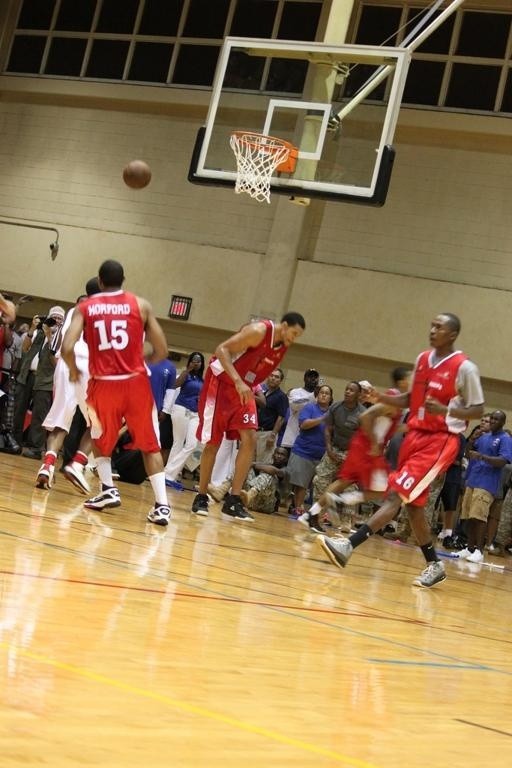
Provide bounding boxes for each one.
[193,355,201,363]
[36,315,56,329]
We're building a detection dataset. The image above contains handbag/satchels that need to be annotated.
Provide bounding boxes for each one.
[0,424,21,454]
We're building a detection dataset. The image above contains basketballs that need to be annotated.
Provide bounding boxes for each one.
[123,160,151,188]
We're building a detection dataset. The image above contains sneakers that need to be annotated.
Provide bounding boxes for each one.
[432,523,507,562]
[412,557,447,588]
[318,531,353,569]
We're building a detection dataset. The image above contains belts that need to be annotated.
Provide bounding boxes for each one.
[257,427,273,431]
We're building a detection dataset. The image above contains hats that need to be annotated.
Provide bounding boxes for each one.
[304,368,319,377]
[47,306,65,322]
[358,381,373,394]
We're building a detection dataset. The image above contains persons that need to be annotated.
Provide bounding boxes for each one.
[316,311,484,590]
[116,350,512,563]
[1,292,88,474]
[60,258,169,527]
[33,277,101,494]
[190,313,306,529]
